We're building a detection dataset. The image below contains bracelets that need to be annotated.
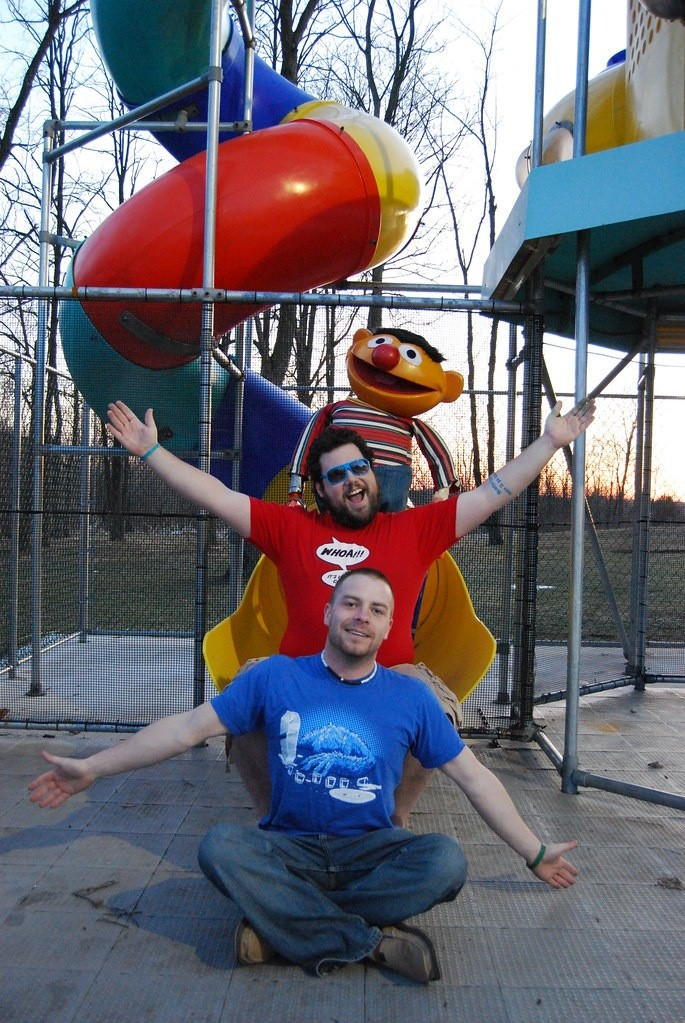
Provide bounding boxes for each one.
[526,844,546,871]
[140,443,162,462]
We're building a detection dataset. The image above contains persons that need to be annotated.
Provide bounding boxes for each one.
[27,568,579,982]
[106,396,597,829]
[288,329,464,508]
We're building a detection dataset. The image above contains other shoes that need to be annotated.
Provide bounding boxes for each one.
[374,922,443,983]
[235,915,278,966]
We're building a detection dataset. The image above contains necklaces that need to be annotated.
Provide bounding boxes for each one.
[321,649,378,685]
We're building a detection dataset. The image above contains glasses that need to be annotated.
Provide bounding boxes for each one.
[319,458,370,485]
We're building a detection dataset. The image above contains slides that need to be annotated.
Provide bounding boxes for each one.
[46,0,501,706]
